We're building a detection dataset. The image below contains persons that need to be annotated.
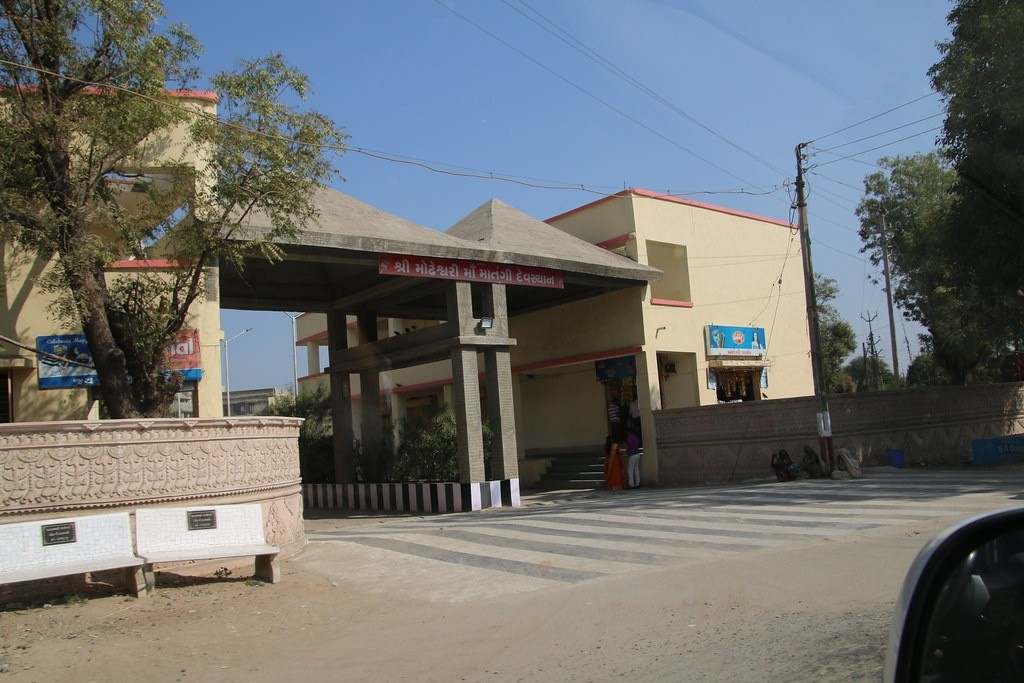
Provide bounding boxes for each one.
[42,344,90,369]
[607,398,623,444]
[830,446,861,479]
[627,394,642,447]
[623,427,642,488]
[770,447,798,480]
[752,332,763,349]
[603,436,622,491]
[799,442,823,479]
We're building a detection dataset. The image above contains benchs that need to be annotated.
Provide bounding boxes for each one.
[0,512,149,598]
[133,503,281,596]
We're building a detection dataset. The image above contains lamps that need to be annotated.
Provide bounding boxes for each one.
[478,317,493,329]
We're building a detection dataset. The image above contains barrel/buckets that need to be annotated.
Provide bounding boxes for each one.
[887,448,904,468]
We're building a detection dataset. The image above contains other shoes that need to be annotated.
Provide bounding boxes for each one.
[624,485,634,490]
[635,486,640,489]
